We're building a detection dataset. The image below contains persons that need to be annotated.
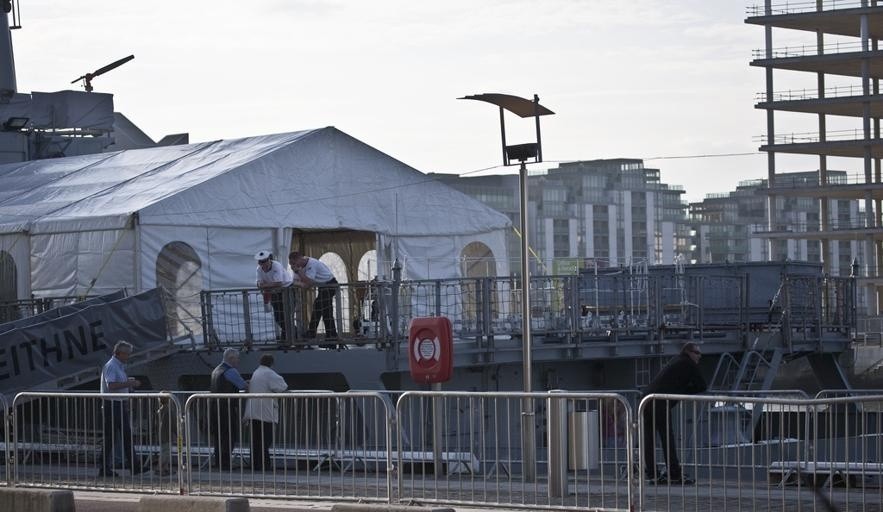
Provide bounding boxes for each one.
[289,252,339,351]
[99,339,150,477]
[256,249,304,345]
[639,341,707,484]
[209,347,248,472]
[244,358,288,473]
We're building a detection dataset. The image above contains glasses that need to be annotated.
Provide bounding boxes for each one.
[690,350,699,354]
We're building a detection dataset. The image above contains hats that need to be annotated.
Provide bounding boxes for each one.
[254,251,269,263]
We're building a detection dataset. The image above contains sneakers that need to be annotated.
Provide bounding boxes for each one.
[648,476,695,486]
[131,466,149,475]
[99,470,118,477]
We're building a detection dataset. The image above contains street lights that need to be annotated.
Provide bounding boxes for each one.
[455,93,556,484]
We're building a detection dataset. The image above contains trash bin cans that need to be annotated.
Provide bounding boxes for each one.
[548,389,598,496]
